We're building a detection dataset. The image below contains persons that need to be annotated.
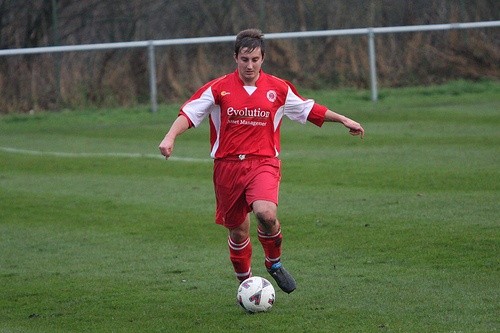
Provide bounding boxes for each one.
[158,29,365,296]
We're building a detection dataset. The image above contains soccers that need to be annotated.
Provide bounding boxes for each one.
[237,276,276,313]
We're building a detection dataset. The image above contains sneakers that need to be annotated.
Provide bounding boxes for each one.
[266,258,297,295]
